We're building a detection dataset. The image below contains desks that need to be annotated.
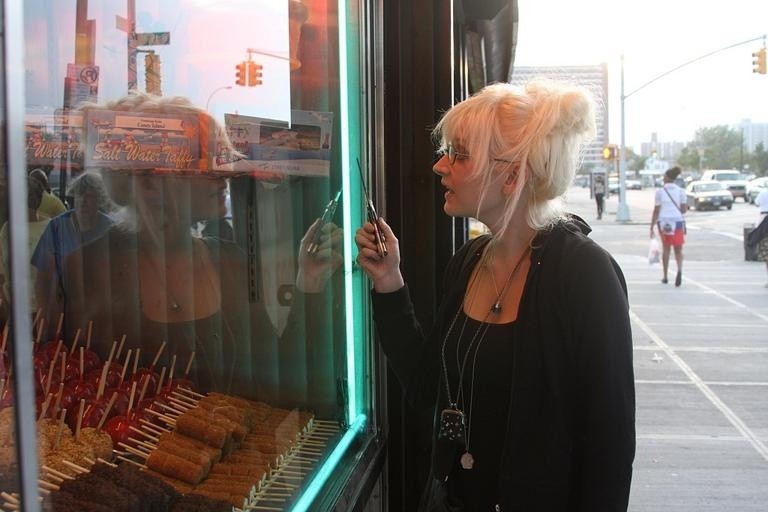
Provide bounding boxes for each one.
[205,84,233,115]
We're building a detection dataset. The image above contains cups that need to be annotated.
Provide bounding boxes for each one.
[438,141,515,168]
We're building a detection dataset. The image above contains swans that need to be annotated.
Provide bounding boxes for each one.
[0,308,196,455]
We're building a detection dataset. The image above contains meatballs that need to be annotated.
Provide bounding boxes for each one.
[356,156,388,259]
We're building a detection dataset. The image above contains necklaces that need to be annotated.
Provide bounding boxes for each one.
[169,302,182,309]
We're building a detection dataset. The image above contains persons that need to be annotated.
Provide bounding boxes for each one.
[31,174,113,345]
[67,94,344,410]
[0,171,64,342]
[355,77,636,511]
[649,166,688,286]
[594,176,605,220]
[754,181,768,221]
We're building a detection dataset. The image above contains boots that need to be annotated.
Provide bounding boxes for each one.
[699,168,747,203]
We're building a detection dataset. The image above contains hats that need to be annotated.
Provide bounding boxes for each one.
[682,179,734,215]
[743,175,767,205]
[571,174,691,197]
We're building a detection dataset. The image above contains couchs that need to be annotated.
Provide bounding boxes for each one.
[749,47,766,76]
[601,145,617,160]
[233,60,246,88]
[247,60,263,86]
